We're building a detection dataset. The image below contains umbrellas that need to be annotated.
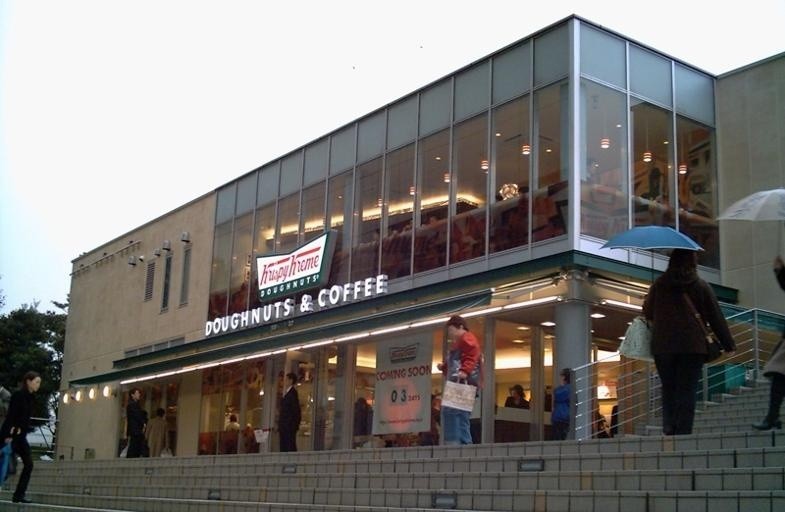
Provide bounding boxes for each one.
[599,225,701,285]
[715,188,784,258]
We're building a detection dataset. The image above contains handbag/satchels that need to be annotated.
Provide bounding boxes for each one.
[618,316,655,362]
[440,380,477,411]
[0,442,11,487]
[705,332,720,360]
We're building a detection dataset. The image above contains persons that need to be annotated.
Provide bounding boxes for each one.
[551,367,571,439]
[501,382,552,412]
[225,413,252,432]
[277,373,301,452]
[126,386,170,458]
[350,398,372,435]
[436,315,484,443]
[0,370,41,503]
[641,249,736,434]
[750,255,785,430]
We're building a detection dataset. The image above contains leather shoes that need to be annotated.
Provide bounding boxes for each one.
[11,495,32,503]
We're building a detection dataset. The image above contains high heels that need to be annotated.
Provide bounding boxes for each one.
[751,417,783,430]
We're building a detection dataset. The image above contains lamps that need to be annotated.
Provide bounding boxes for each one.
[642,112,653,164]
[677,119,686,176]
[601,108,610,150]
[62,385,118,404]
[501,182,520,200]
[521,98,531,155]
[378,198,383,206]
[444,173,449,183]
[410,186,415,196]
[480,118,489,169]
